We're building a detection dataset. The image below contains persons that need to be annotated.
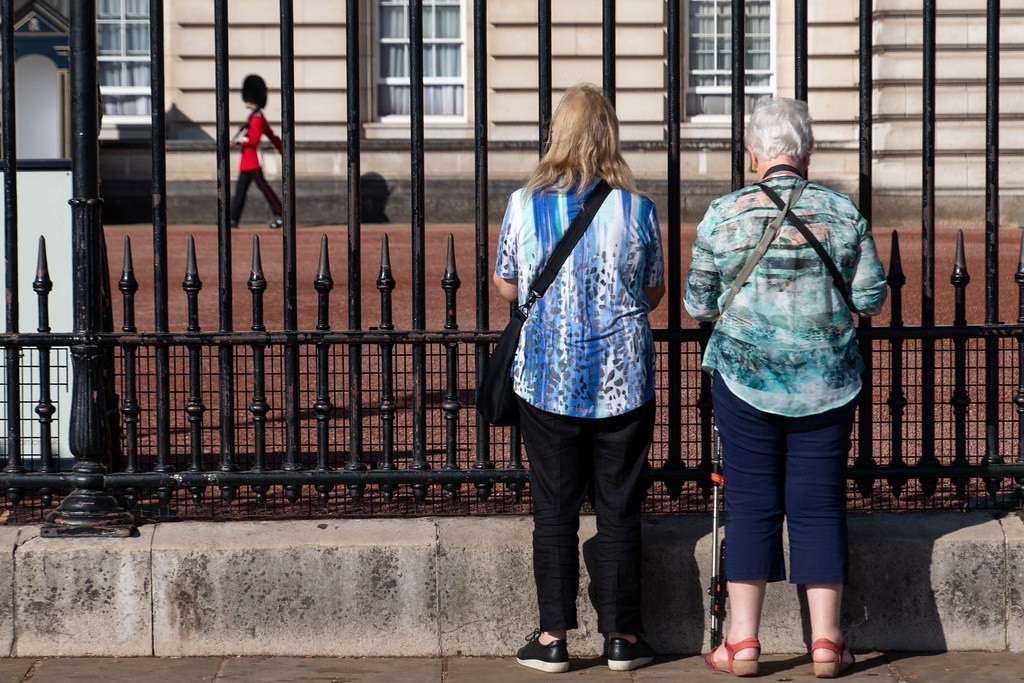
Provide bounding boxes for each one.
[492,82,665,673]
[214,73,288,228]
[682,97,888,678]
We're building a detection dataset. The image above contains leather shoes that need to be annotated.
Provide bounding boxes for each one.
[607,633,654,671]
[515,634,570,673]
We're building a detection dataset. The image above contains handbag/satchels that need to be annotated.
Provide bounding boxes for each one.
[477,298,531,427]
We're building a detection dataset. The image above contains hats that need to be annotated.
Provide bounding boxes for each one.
[241,74,268,108]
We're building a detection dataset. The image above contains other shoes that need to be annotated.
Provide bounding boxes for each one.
[268,218,283,228]
[215,217,239,228]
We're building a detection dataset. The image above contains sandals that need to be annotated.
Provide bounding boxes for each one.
[705,638,761,677]
[812,637,856,677]
